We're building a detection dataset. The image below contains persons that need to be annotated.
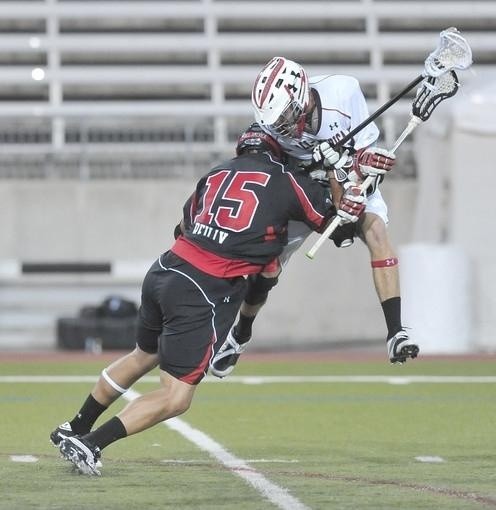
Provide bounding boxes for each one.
[50,121,344,476]
[210,56,419,377]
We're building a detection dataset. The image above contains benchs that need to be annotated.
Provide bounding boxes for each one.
[0,0,496,144]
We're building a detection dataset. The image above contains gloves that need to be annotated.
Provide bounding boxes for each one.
[310,141,348,180]
[353,147,396,177]
[337,186,367,223]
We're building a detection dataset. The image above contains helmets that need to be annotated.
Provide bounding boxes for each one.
[252,56,308,140]
[236,122,287,162]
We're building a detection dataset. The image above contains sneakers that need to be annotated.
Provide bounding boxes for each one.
[50,423,102,474]
[211,330,251,379]
[386,331,419,365]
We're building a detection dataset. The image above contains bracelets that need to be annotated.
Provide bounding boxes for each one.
[329,178,336,180]
